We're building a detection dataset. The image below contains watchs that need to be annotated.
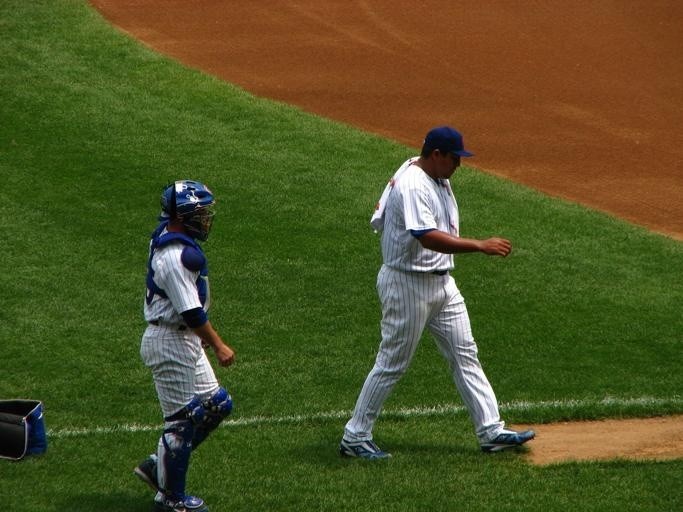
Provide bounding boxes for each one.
[339,127,535,459]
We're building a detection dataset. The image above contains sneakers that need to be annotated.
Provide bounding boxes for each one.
[339,437,392,459]
[480,430,535,452]
[134,458,209,512]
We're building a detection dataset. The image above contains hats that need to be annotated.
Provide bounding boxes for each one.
[425,127,475,156]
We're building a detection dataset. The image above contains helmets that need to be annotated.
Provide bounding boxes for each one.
[158,180,215,221]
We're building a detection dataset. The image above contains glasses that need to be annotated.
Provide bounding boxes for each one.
[452,156,459,164]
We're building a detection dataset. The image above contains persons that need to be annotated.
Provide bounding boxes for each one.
[134,180,235,512]
